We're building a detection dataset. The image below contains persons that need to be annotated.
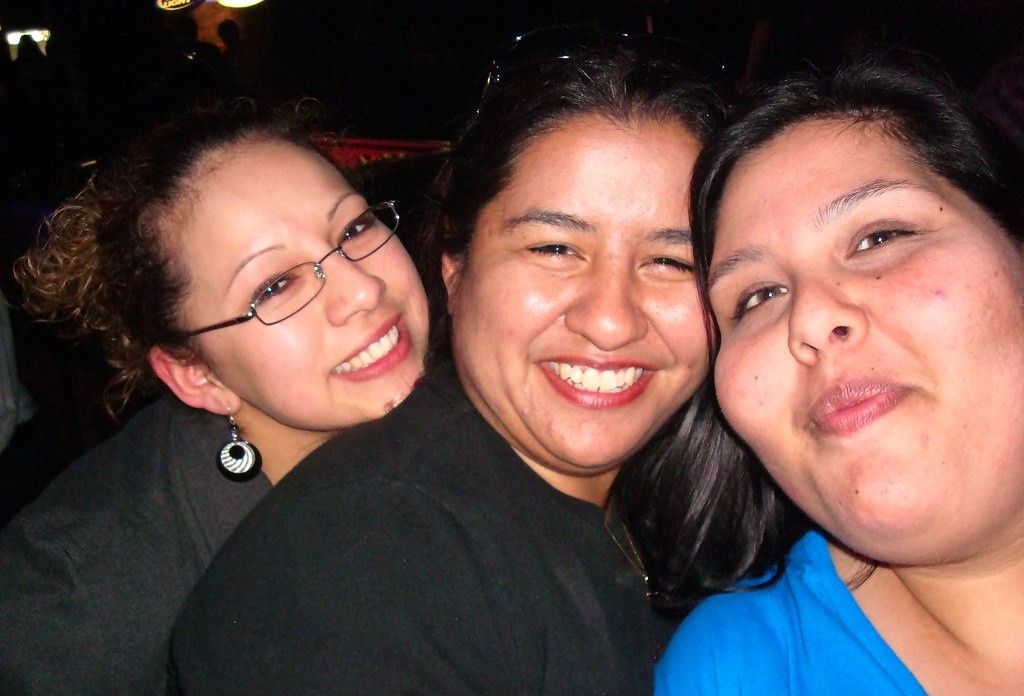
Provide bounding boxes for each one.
[169,41,813,696]
[651,50,1024,696]
[0,98,430,696]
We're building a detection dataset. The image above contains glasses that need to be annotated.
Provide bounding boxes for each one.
[176,198,400,341]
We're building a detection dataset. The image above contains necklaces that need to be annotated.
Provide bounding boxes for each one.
[605,520,651,601]
